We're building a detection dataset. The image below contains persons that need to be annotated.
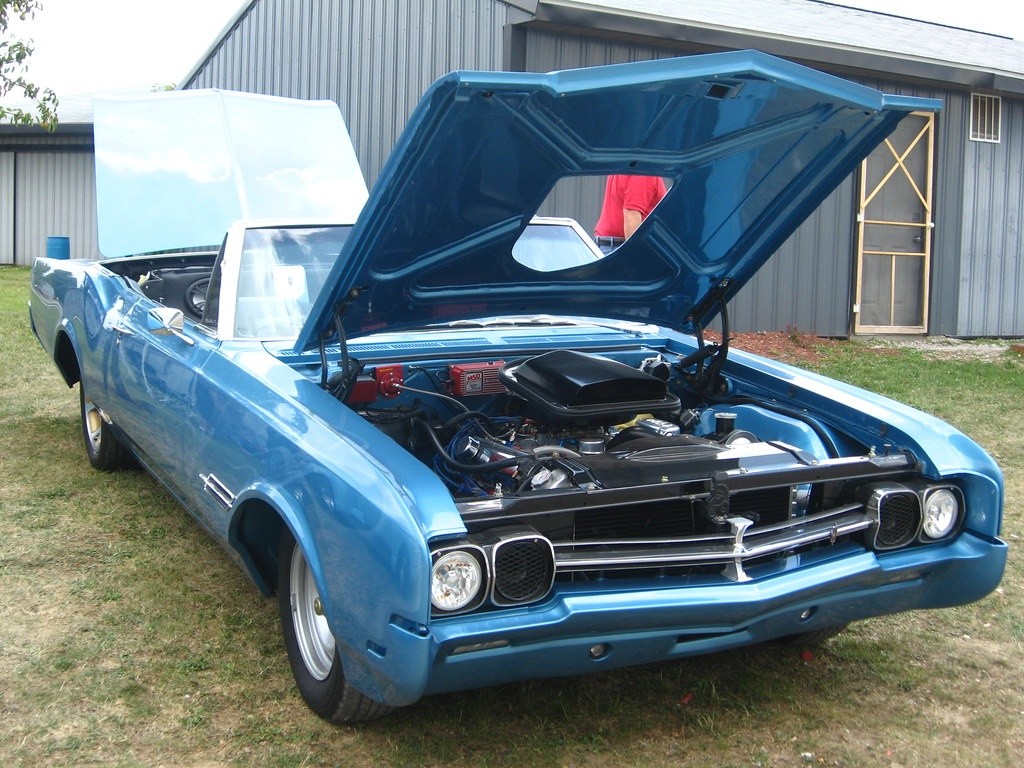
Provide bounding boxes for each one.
[594,174,667,255]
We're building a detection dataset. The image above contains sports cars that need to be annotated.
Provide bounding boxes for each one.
[28,46,1007,723]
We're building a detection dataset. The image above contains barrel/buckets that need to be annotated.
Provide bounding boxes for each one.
[46,237,70,259]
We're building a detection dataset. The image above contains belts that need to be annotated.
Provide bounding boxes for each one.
[595,240,626,247]
